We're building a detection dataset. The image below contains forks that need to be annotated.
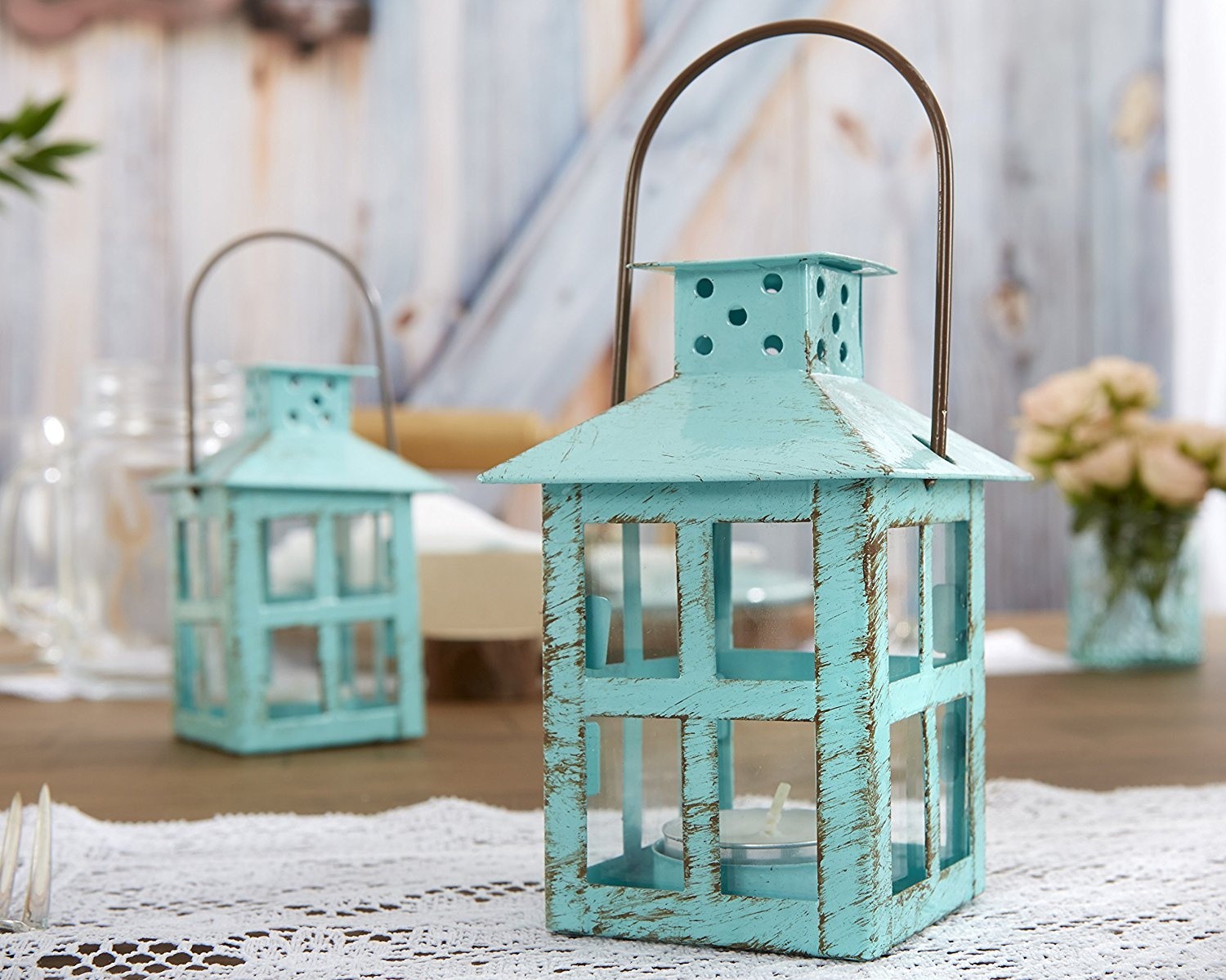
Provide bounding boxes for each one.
[0,782,53,932]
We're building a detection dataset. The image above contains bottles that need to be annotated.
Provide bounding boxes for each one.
[0,358,253,702]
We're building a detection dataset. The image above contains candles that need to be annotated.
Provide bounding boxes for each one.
[661,782,816,865]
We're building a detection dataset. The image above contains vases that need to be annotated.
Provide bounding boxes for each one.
[1065,530,1209,672]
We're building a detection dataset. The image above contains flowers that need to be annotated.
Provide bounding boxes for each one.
[1008,354,1226,657]
